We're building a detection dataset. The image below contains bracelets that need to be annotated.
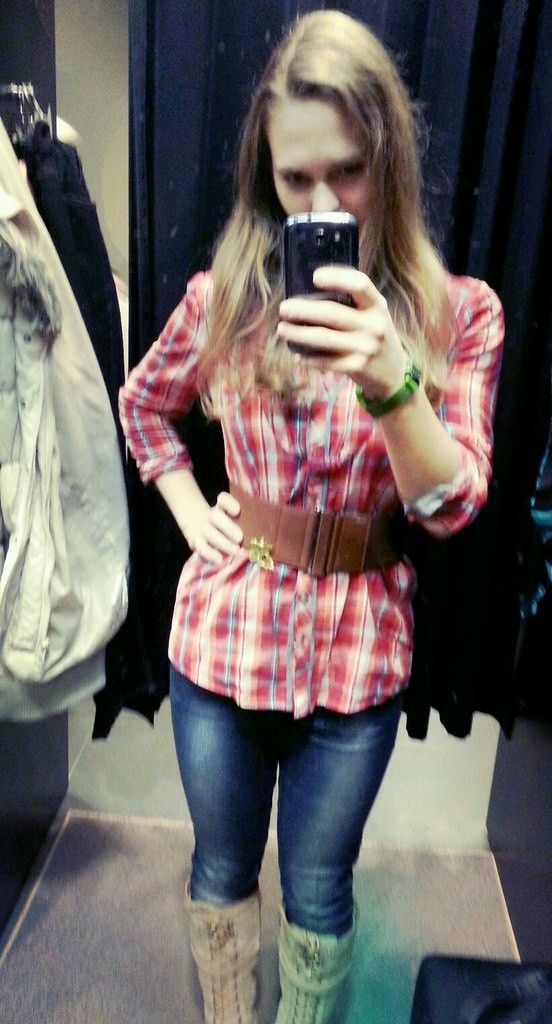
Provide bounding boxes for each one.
[354,341,422,420]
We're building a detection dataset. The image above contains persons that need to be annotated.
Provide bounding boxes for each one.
[119,9,505,1024]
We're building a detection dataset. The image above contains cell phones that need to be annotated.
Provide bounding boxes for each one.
[282,213,359,358]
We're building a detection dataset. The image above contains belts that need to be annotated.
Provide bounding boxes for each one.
[226,484,404,578]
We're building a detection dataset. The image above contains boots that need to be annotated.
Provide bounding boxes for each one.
[183,879,261,1024]
[271,898,355,1024]
